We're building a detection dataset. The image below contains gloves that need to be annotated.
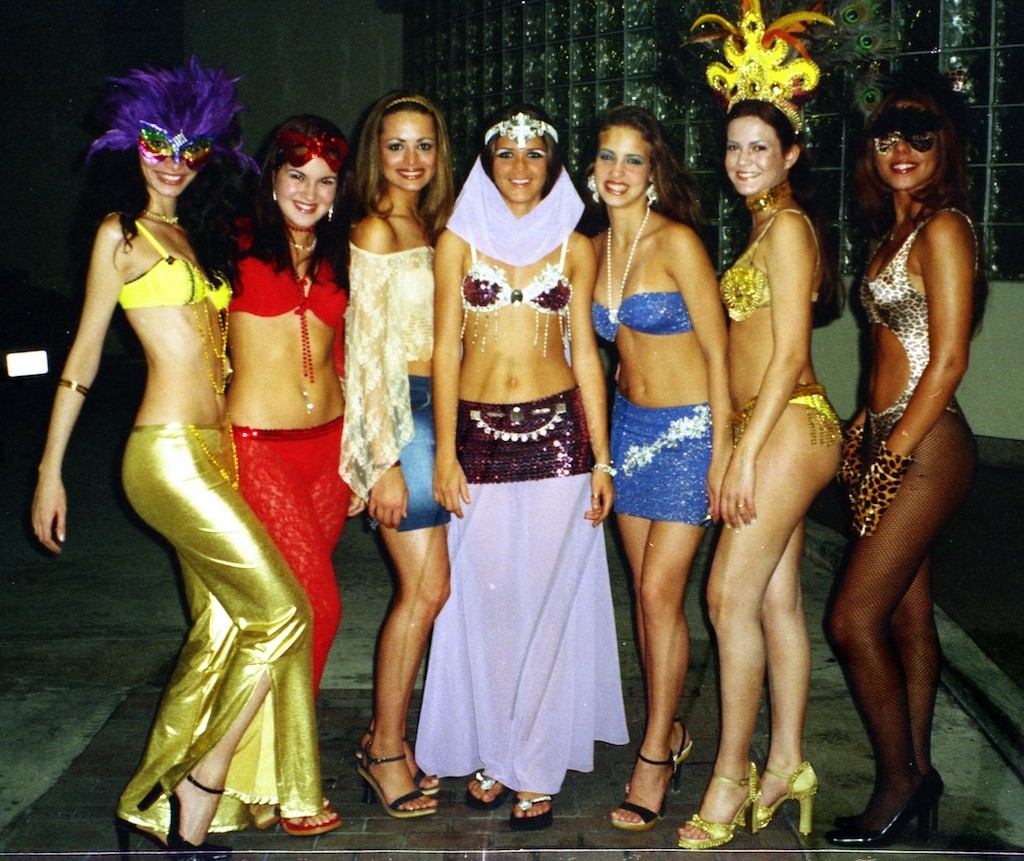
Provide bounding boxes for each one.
[839,425,870,493]
[849,441,915,536]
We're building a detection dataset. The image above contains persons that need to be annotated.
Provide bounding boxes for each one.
[567,106,731,832]
[677,89,842,851]
[823,85,979,850]
[410,105,628,829]
[320,90,455,822]
[218,113,368,702]
[31,85,313,860]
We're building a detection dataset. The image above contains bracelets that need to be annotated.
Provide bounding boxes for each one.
[57,378,90,398]
[590,463,617,476]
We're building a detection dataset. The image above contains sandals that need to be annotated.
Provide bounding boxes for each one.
[275,816,343,836]
[465,772,513,811]
[510,795,553,831]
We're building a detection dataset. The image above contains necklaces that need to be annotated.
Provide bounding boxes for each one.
[142,208,179,224]
[606,208,652,320]
[284,221,317,251]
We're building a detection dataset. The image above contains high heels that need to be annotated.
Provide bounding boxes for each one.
[735,760,819,833]
[116,815,180,861]
[825,775,933,848]
[137,771,233,861]
[610,746,676,831]
[355,726,442,805]
[624,717,694,797]
[833,766,945,831]
[677,761,763,850]
[355,741,437,818]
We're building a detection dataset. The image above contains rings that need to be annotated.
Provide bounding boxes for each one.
[738,504,745,510]
